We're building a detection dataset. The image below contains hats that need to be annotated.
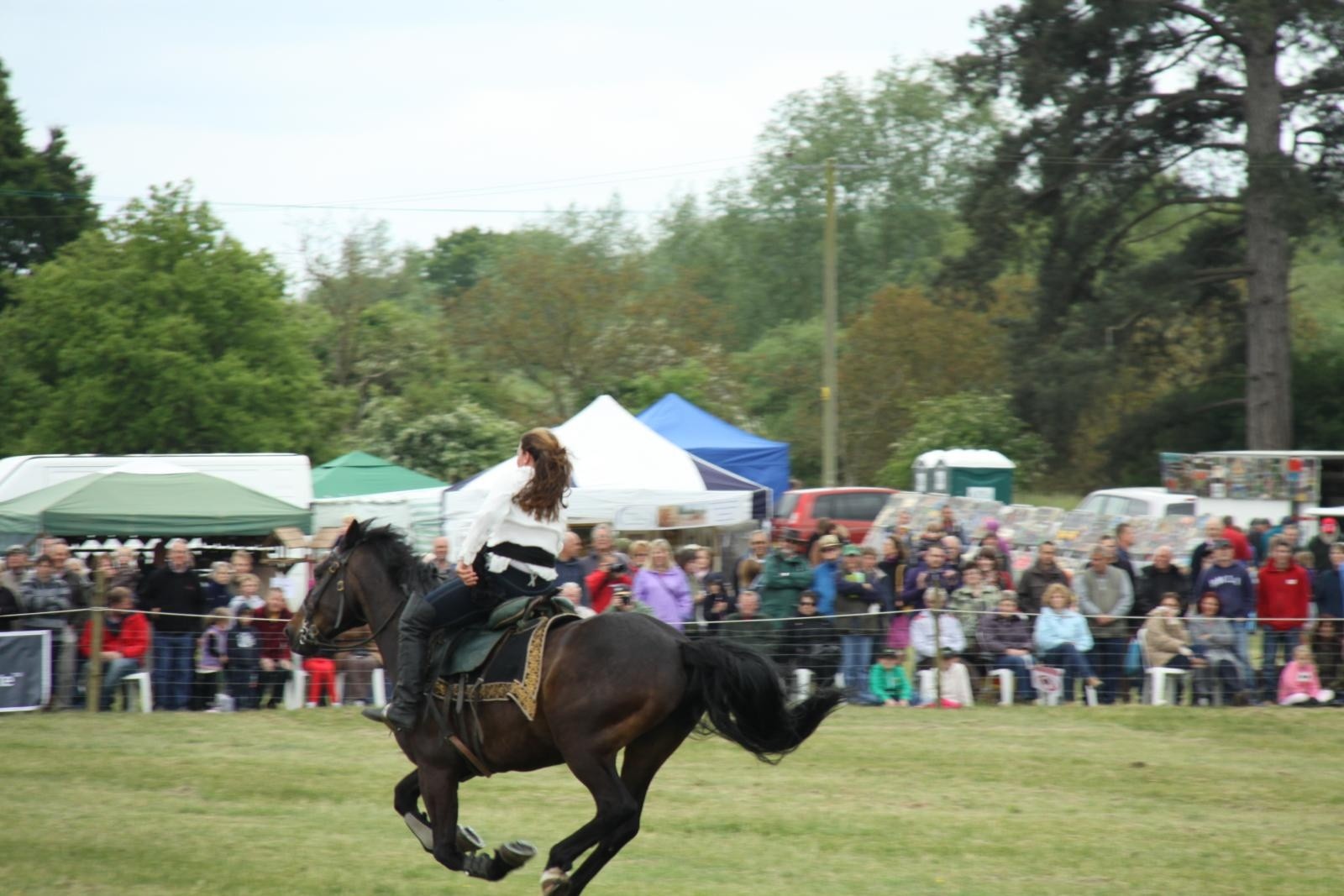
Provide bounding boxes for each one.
[939,647,955,659]
[778,529,809,544]
[995,590,1019,602]
[882,648,899,660]
[843,544,861,558]
[5,544,26,554]
[1322,518,1338,533]
[818,534,843,549]
[1213,539,1231,551]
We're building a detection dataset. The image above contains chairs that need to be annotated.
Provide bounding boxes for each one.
[794,667,846,705]
[279,649,390,709]
[1137,629,1191,708]
[975,633,1015,708]
[115,670,155,715]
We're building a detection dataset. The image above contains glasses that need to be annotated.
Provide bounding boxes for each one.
[752,541,765,546]
[822,548,835,552]
[785,539,799,544]
[799,601,815,607]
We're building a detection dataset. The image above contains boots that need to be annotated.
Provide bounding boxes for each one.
[360,589,440,734]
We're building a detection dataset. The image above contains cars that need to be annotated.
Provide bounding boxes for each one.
[767,485,900,557]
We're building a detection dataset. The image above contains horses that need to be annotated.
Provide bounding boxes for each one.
[282,516,865,896]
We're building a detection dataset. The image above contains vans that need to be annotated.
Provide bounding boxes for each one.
[1073,487,1294,533]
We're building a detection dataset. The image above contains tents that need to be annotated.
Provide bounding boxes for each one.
[0,392,791,567]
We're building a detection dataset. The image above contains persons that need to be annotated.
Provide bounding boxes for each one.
[363,429,575,728]
[558,517,1344,706]
[0,535,386,711]
[424,537,461,579]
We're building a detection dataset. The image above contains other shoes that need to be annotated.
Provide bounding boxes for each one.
[1086,677,1105,690]
[1232,689,1251,706]
